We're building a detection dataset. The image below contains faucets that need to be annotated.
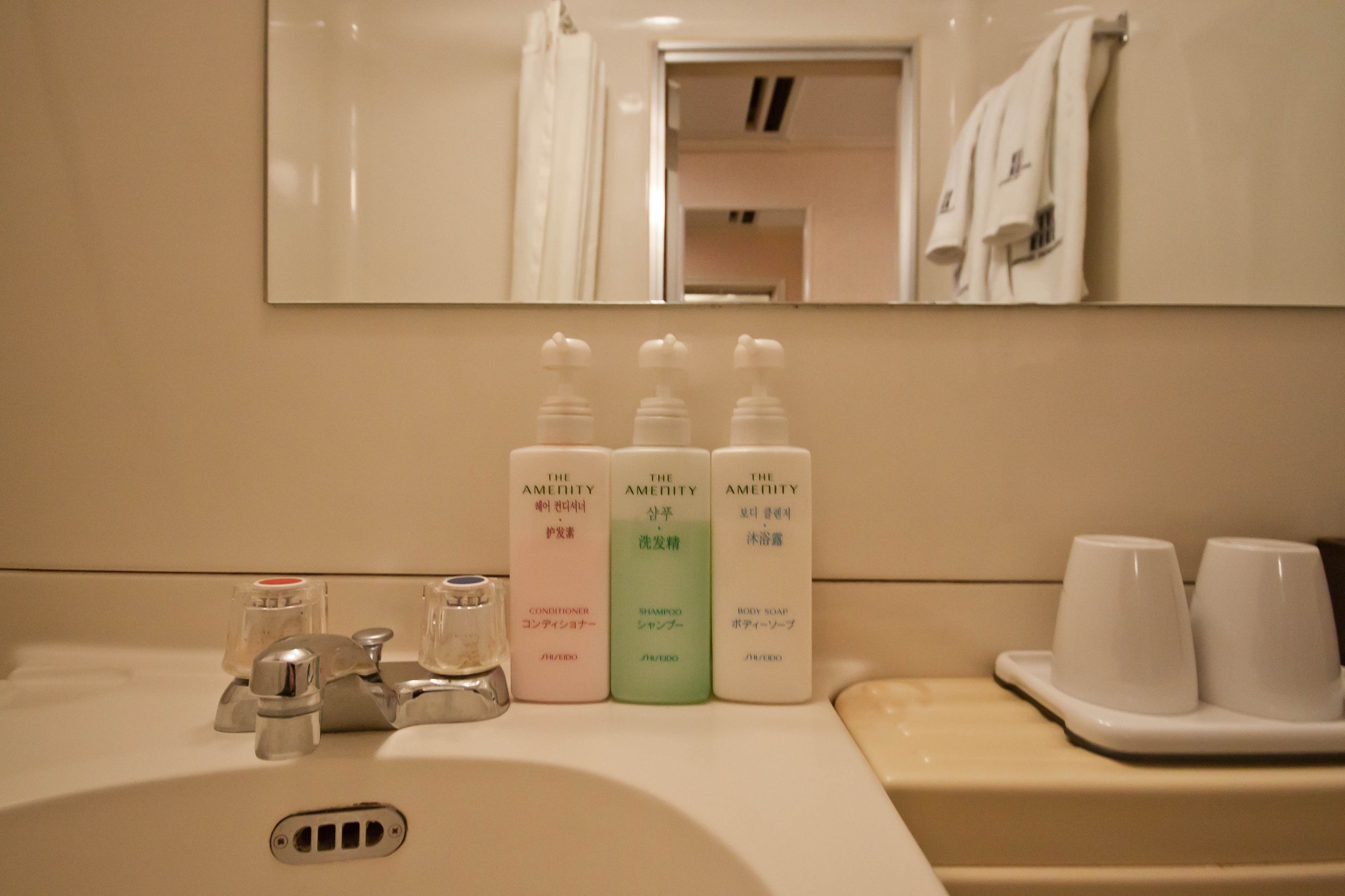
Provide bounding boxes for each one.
[248,631,397,763]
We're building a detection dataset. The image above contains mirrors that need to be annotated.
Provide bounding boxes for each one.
[266,0,1344,306]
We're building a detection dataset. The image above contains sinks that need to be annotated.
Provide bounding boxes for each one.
[0,752,776,896]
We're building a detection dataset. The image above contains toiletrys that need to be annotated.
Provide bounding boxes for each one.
[606,334,712,706]
[506,331,614,706]
[708,331,814,708]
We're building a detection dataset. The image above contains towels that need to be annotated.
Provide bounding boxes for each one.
[924,15,1112,308]
